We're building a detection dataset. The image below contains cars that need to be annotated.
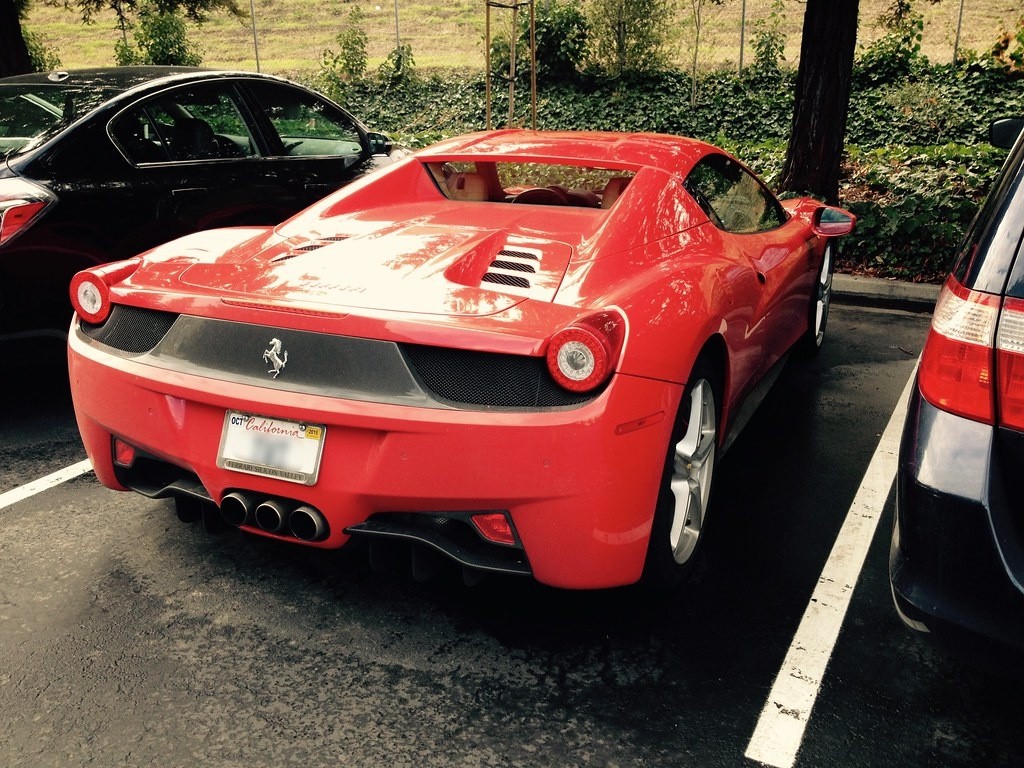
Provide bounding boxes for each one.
[1,66,393,416]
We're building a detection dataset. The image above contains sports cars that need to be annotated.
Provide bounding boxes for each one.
[66,126,854,593]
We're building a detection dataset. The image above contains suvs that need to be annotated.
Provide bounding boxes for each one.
[885,124,1024,668]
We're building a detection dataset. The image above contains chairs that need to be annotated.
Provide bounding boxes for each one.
[113,113,248,162]
[601,177,634,210]
[447,171,494,204]
